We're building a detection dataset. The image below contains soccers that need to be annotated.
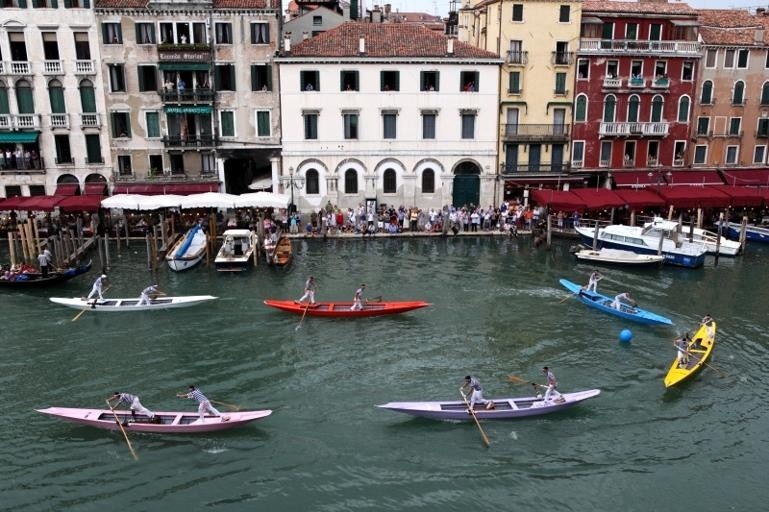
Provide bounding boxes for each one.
[619,329,633,343]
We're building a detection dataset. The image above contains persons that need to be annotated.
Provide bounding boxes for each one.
[135,282,166,305]
[542,364,565,405]
[88,274,107,302]
[675,337,690,362]
[178,35,188,43]
[297,276,318,304]
[106,391,157,421]
[160,78,212,108]
[586,271,607,291]
[461,375,493,412]
[610,291,635,313]
[350,284,365,312]
[178,386,231,423]
[703,314,716,340]
[2,147,41,173]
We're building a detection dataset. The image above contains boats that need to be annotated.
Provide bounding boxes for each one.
[558,278,673,330]
[0,257,94,290]
[371,387,603,421]
[165,223,208,273]
[263,297,435,318]
[271,236,293,271]
[32,406,274,435]
[663,319,718,390]
[573,216,767,267]
[213,227,260,274]
[48,294,219,313]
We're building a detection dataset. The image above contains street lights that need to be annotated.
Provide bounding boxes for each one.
[647,163,672,189]
[278,165,305,213]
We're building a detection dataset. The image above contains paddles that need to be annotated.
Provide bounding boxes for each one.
[673,342,726,377]
[510,375,549,389]
[74,286,111,319]
[359,295,382,304]
[105,399,136,460]
[461,390,489,446]
